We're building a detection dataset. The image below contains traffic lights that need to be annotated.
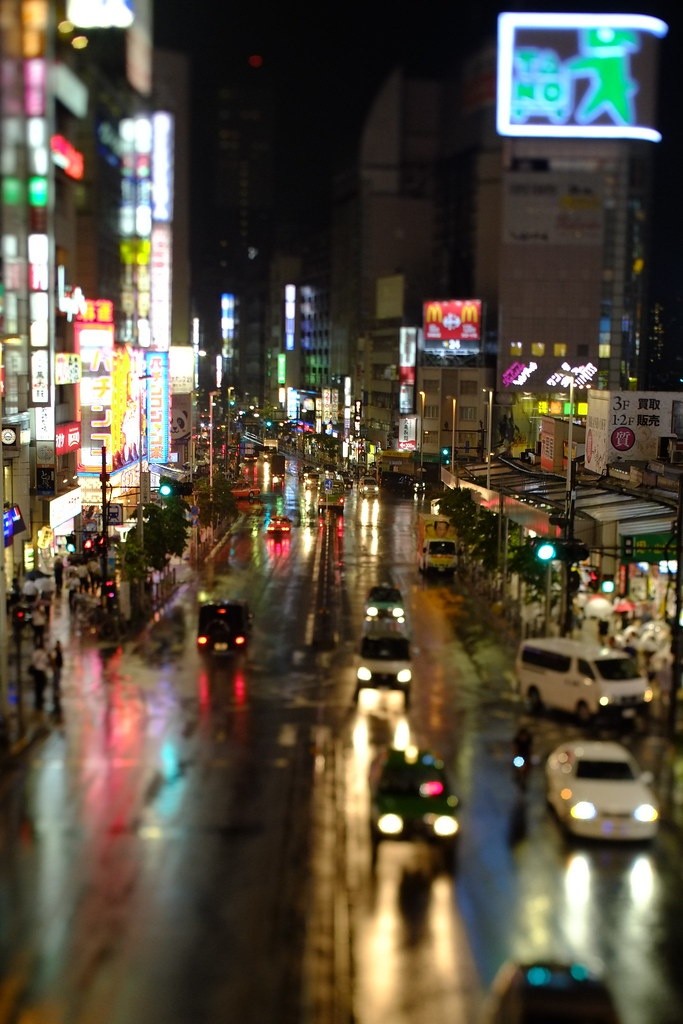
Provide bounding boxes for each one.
[530,541,589,563]
[159,483,194,497]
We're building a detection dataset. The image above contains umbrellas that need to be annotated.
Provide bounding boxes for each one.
[24,569,45,578]
[584,598,670,636]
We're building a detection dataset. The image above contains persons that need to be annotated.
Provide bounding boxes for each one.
[352,458,357,470]
[31,640,63,706]
[10,552,101,624]
[31,606,46,646]
[513,725,533,788]
[573,590,659,684]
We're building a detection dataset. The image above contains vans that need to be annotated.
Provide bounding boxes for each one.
[515,636,645,722]
[418,536,458,574]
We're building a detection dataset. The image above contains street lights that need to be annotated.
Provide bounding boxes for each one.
[446,395,456,475]
[482,387,493,490]
[418,390,426,495]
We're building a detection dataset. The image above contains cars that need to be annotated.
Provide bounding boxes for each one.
[351,583,413,711]
[195,599,250,653]
[230,479,260,499]
[368,747,459,858]
[268,454,432,514]
[544,737,659,845]
[266,515,294,534]
